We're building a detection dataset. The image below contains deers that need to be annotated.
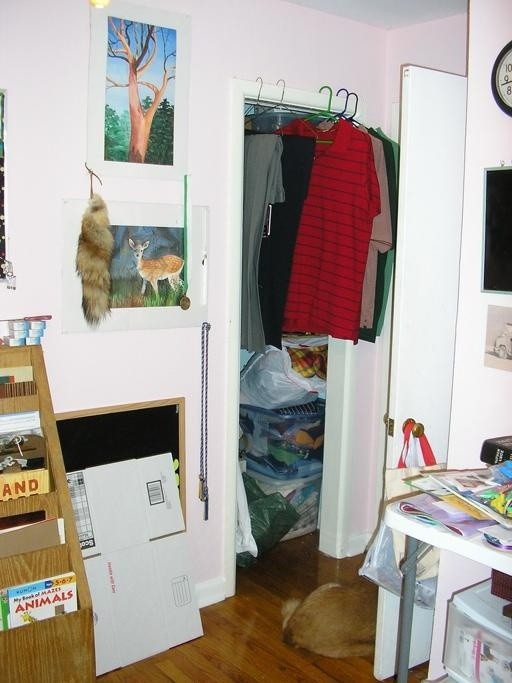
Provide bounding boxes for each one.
[125,239,185,301]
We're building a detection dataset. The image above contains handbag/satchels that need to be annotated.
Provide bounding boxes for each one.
[357,423,440,614]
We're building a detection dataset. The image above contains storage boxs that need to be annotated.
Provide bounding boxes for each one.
[241,471,320,543]
[443,578,512,683]
[239,403,323,479]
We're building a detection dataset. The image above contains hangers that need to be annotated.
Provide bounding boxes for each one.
[244,77,370,141]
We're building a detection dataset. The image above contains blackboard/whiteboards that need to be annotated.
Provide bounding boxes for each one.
[54,396,186,560]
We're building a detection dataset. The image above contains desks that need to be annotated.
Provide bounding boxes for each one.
[384,494,512,683]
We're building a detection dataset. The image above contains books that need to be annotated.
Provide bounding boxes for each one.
[400,467,512,539]
[2,366,77,634]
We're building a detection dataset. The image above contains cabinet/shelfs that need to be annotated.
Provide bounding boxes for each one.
[0,346,97,682]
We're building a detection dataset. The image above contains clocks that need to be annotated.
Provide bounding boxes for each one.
[491,41,512,117]
